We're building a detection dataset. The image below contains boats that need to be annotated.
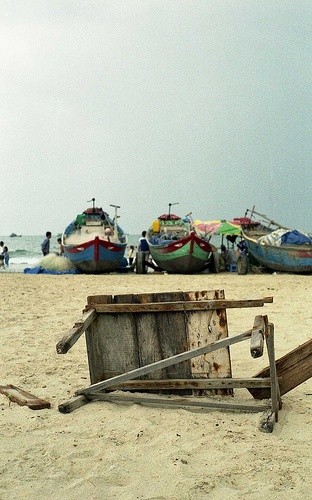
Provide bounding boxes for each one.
[227,205,312,274]
[60,198,129,274]
[145,203,213,275]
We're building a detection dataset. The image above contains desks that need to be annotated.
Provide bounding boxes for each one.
[57,289,282,431]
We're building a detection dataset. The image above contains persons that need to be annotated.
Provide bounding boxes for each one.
[139,231,151,273]
[0,240,9,270]
[221,244,236,264]
[54,238,64,256]
[127,245,134,265]
[41,232,52,256]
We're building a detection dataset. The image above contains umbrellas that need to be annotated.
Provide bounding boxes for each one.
[193,219,244,257]
